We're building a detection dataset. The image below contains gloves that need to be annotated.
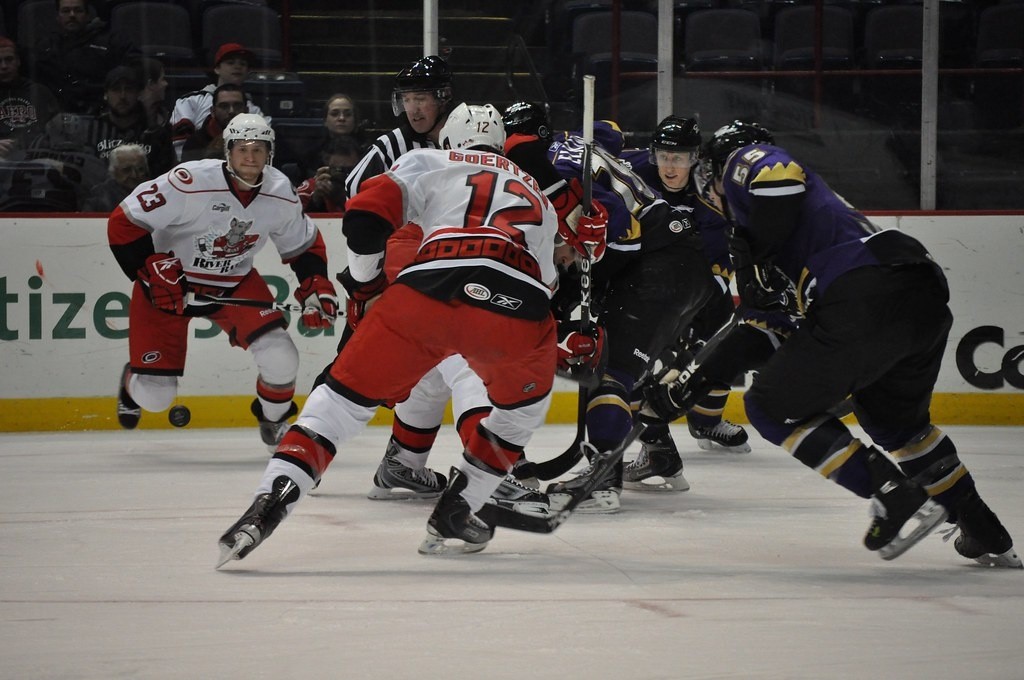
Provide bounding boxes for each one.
[337,266,388,332]
[138,249,192,316]
[728,244,798,312]
[293,275,339,329]
[556,318,610,389]
[638,345,725,425]
[554,178,609,264]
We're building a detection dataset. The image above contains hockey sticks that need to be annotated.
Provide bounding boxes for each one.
[475,305,743,536]
[531,74,595,481]
[189,292,349,319]
[630,288,715,391]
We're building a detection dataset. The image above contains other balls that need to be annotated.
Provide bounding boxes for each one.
[168,405,191,427]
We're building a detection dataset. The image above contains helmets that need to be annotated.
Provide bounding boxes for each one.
[647,115,701,168]
[47,112,89,152]
[439,102,507,154]
[710,119,775,160]
[694,158,714,197]
[391,55,451,116]
[501,102,548,137]
[222,113,276,174]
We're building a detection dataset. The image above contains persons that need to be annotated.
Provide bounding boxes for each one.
[107,111,339,454]
[616,115,799,455]
[637,119,1024,573]
[365,134,610,534]
[500,99,731,517]
[0,0,382,213]
[307,53,453,391]
[216,101,559,572]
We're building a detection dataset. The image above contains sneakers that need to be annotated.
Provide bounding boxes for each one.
[621,432,690,492]
[416,465,493,561]
[476,476,558,534]
[937,506,1024,569]
[250,397,299,454]
[214,475,300,570]
[543,442,622,514]
[686,416,751,454]
[366,437,447,501]
[116,363,143,430]
[833,444,945,559]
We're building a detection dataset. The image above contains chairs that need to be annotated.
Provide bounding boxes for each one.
[522,0,1023,173]
[0,0,364,216]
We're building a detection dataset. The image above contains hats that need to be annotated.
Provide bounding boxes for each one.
[213,43,255,68]
[0,37,14,48]
[104,65,141,90]
